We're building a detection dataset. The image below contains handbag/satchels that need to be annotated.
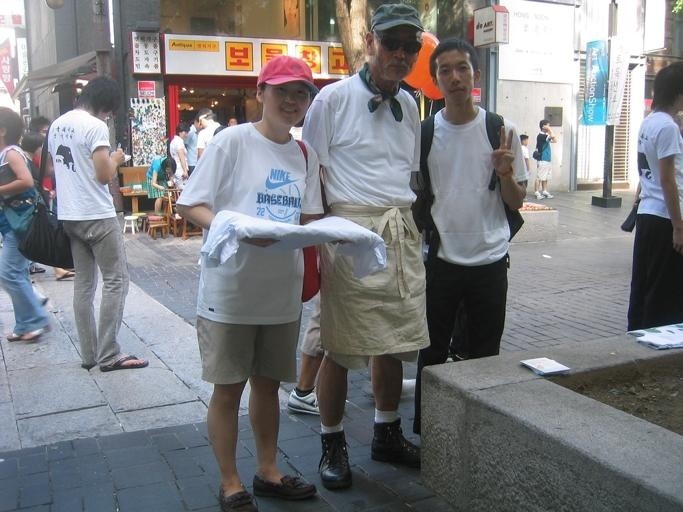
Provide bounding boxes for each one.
[18,125,74,269]
[532,142,549,160]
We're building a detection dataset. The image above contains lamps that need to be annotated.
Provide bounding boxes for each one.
[51,82,83,93]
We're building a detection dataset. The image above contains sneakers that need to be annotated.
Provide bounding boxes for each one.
[7,331,23,342]
[21,325,52,340]
[287,386,321,416]
[542,190,554,199]
[534,192,545,200]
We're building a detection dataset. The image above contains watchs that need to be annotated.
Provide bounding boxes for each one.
[493,166,514,177]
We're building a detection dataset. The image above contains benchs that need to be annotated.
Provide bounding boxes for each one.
[118,166,150,213]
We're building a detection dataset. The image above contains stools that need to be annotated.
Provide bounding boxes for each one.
[123,215,140,235]
[148,220,167,236]
[150,223,168,240]
[131,212,147,232]
[147,215,164,235]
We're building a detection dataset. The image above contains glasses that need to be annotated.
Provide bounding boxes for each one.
[378,34,422,54]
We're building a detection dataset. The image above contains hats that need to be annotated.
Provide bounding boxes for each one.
[370,4,426,34]
[256,55,318,94]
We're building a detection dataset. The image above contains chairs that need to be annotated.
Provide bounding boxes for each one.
[165,188,183,237]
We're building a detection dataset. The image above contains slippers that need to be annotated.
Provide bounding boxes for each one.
[100,355,148,372]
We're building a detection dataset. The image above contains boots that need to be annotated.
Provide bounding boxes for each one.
[371,417,420,468]
[318,430,352,490]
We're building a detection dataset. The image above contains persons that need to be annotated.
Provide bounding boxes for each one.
[169,122,190,190]
[183,115,203,178]
[288,246,416,416]
[196,108,221,165]
[0,107,55,344]
[37,74,149,373]
[532,119,558,200]
[144,154,177,213]
[411,37,529,439]
[226,115,238,129]
[29,116,56,214]
[627,62,683,332]
[173,54,354,512]
[287,4,434,492]
[519,134,531,172]
[21,130,45,192]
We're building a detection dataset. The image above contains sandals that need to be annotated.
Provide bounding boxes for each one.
[217,482,260,512]
[252,472,316,500]
